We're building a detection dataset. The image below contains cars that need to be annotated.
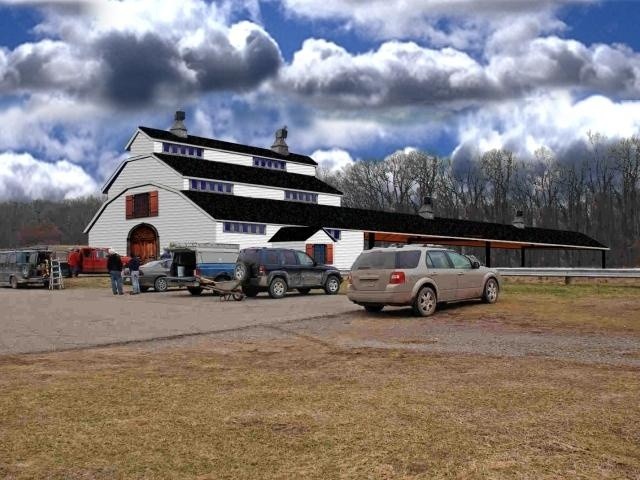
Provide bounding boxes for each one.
[122,259,185,291]
[80,247,132,273]
[346,243,500,316]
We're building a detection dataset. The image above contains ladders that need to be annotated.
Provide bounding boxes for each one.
[49,257,65,291]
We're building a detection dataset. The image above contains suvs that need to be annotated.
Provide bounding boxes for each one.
[233,246,344,299]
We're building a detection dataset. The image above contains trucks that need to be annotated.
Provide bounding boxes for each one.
[0,249,53,288]
[171,243,240,295]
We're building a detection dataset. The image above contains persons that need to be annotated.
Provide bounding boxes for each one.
[107,248,124,294]
[126,252,141,294]
[67,248,81,278]
